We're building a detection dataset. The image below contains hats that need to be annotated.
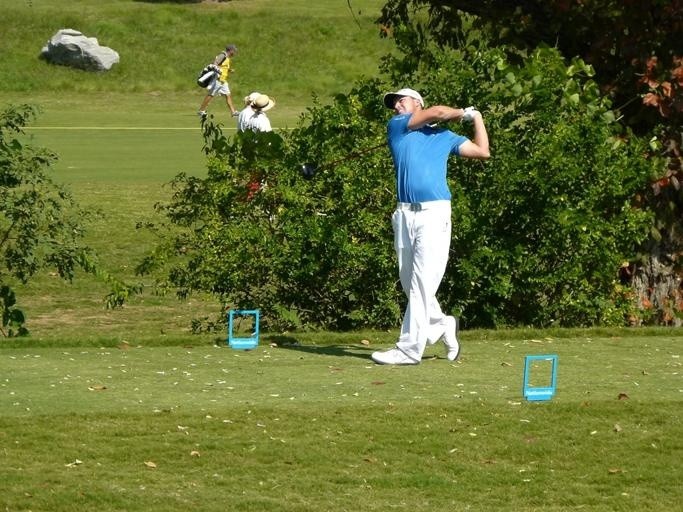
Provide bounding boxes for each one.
[248,92,261,100]
[384,88,424,109]
[250,95,275,112]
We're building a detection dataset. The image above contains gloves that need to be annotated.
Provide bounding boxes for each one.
[462,106,479,120]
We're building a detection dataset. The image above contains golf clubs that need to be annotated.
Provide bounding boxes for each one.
[302,106,475,180]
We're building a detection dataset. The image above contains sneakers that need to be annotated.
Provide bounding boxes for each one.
[442,316,460,361]
[372,348,419,365]
[232,112,239,116]
[197,111,207,116]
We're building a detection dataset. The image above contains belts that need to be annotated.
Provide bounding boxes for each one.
[397,200,451,211]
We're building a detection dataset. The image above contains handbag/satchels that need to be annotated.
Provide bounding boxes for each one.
[197,71,217,88]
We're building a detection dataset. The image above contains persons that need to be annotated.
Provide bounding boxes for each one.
[238,95,272,133]
[243,92,260,108]
[197,45,239,117]
[370,88,490,365]
